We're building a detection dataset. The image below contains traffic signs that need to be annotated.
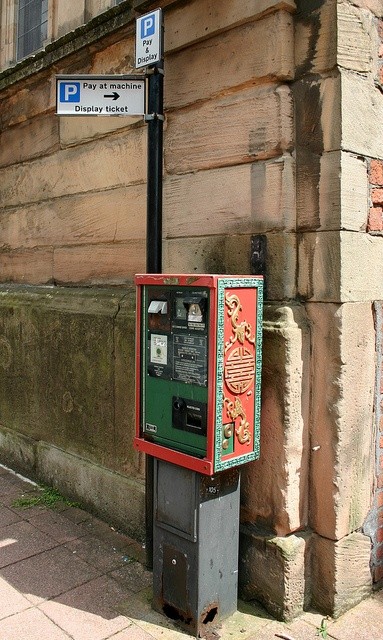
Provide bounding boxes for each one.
[56,74,147,118]
[134,7,164,70]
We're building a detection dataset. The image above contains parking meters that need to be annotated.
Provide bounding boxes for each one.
[133,272,265,476]
